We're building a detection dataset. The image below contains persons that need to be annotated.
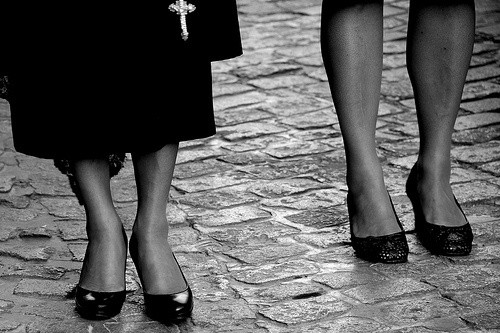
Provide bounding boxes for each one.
[319,0,477,262]
[0,0,241,321]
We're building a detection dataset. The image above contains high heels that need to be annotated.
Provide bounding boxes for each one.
[406,162,475,256]
[344,181,410,262]
[128,228,194,322]
[73,218,128,321]
